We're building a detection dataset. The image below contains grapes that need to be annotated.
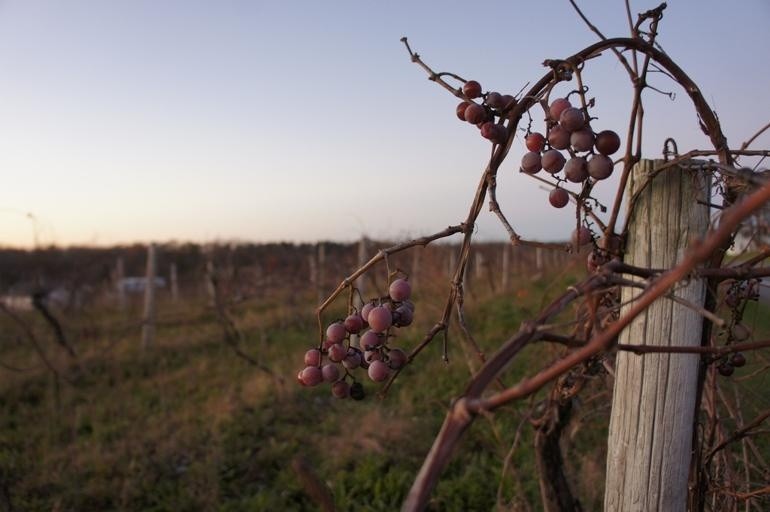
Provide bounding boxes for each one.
[457,80,622,272]
[712,277,753,379]
[296,278,417,401]
